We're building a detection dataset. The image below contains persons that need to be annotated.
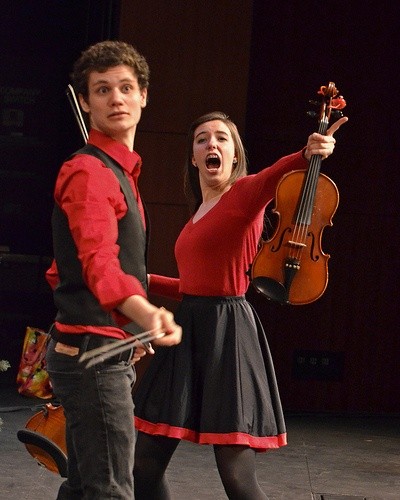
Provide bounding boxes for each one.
[132,112,349,500]
[45,41,182,500]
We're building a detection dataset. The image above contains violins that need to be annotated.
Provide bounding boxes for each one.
[249,82,346,306]
[17,403,68,479]
